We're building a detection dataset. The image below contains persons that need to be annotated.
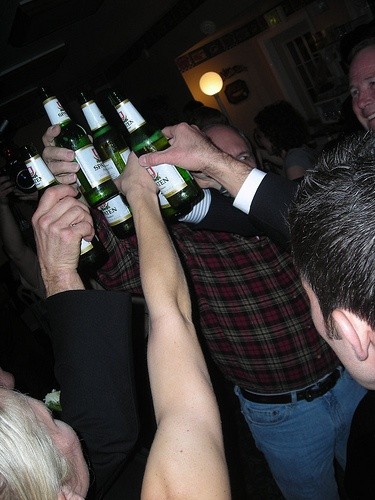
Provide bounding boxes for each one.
[1,148,233,500]
[1,183,157,500]
[0,36,374,500]
[139,120,374,500]
[42,124,368,500]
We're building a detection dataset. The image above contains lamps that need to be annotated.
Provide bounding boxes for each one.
[199,72,234,126]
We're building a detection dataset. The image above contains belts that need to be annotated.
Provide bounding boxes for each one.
[239,364,344,404]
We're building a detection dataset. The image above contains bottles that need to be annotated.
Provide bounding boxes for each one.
[19,142,106,271]
[34,83,116,206]
[107,86,205,212]
[76,89,153,206]
[157,191,192,217]
[97,190,135,239]
